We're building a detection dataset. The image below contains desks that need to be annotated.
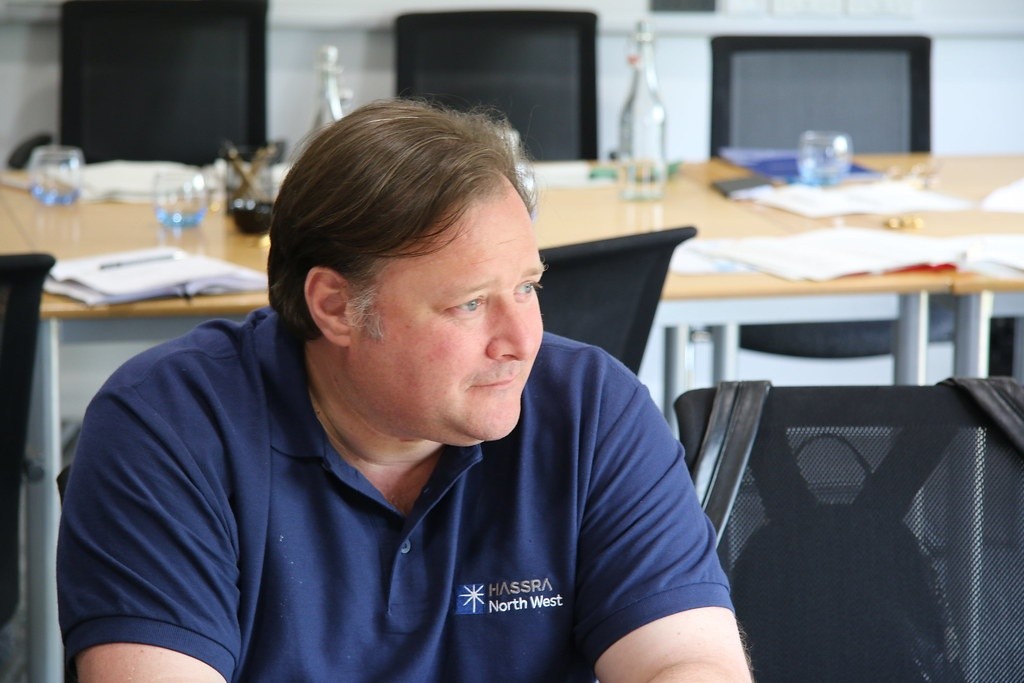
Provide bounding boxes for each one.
[0,150,1024,683]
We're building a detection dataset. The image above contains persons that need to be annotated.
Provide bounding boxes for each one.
[57,99,754,683]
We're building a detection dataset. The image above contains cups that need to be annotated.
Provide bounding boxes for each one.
[27,145,86,208]
[797,130,853,187]
[150,166,205,229]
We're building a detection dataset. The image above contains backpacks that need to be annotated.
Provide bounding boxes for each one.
[674,378,1021,679]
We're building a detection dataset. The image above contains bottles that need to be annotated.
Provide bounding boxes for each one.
[307,44,344,131]
[621,23,665,202]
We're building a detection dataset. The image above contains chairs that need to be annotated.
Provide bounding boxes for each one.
[688,35,961,386]
[60,0,266,165]
[532,226,697,378]
[0,253,56,631]
[669,373,1024,683]
[394,10,599,162]
